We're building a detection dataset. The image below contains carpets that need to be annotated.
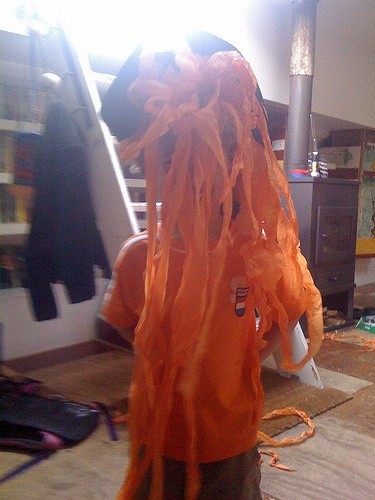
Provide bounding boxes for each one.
[26,347,354,450]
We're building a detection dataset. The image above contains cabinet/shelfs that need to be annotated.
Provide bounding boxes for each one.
[326,128,375,257]
[68,35,163,271]
[0,30,114,362]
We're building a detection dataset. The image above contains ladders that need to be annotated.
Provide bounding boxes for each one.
[60,25,326,399]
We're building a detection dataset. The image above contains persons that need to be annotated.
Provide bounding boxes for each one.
[93,110,313,500]
